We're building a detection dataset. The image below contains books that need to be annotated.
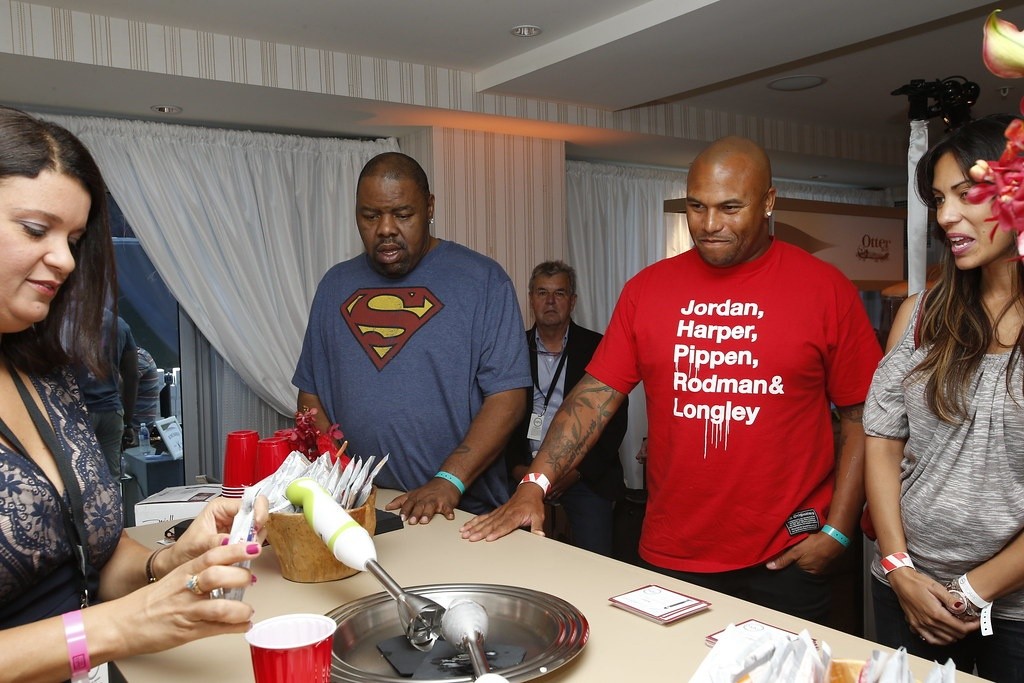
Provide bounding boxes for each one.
[705,619,820,653]
[154,414,185,462]
[608,585,711,625]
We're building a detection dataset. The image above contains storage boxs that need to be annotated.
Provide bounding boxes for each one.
[133,483,223,525]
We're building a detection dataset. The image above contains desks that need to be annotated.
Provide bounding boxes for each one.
[108,488,998,683]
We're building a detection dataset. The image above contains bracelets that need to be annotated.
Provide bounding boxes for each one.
[145,543,176,582]
[878,551,915,578]
[435,471,466,494]
[518,473,552,498]
[60,609,91,675]
[942,573,994,636]
[819,524,850,548]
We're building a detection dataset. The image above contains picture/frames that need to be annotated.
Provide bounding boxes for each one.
[156,416,182,460]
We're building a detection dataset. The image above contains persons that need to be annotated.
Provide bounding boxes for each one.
[57,270,159,484]
[507,260,630,554]
[459,134,882,620]
[291,151,534,526]
[0,107,266,683]
[863,115,1024,683]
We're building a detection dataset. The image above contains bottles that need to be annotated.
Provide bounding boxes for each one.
[138,423,151,455]
[150,425,159,445]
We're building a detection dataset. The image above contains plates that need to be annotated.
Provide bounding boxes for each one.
[324,583,589,683]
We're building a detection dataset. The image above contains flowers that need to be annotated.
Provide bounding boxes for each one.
[965,9,1024,264]
[282,404,344,462]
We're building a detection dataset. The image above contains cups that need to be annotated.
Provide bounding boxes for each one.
[243,613,337,683]
[221,429,296,498]
